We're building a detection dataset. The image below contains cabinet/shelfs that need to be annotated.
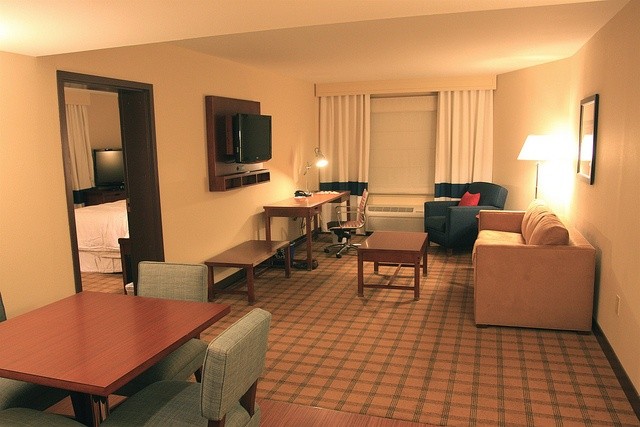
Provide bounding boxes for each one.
[205,95,271,192]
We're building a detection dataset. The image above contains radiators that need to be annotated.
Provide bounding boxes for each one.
[365,204,425,233]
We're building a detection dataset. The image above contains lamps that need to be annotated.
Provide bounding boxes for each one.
[516,134,559,199]
[303,148,327,193]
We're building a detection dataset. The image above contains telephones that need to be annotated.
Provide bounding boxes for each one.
[294,190,313,197]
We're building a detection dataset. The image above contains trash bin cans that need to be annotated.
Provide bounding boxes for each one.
[275,242,296,267]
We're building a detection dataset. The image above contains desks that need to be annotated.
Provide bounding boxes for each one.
[262,190,352,271]
[1,292,231,419]
[206,239,292,306]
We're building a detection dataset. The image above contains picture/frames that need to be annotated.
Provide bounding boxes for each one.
[575,94,598,184]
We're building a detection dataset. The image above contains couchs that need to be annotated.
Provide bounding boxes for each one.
[471,199,595,331]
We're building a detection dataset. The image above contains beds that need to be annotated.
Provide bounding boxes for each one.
[71,200,130,272]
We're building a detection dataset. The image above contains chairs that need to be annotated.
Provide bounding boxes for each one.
[100,307,270,427]
[324,189,369,257]
[1,376,89,420]
[424,182,508,253]
[114,262,207,385]
[0,406,86,427]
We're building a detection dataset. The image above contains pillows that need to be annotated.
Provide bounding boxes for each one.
[457,192,481,207]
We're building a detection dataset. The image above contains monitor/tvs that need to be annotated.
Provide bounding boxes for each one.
[233,113,272,163]
[92,147,127,188]
